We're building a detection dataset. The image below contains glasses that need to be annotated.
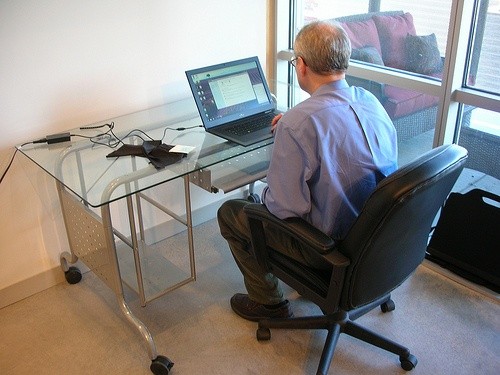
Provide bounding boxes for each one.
[289,58,308,68]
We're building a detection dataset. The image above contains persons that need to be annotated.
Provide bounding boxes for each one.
[217,19,398,322]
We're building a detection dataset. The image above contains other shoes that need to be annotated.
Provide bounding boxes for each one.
[230,293,295,322]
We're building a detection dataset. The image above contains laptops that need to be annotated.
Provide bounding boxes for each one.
[185,56,283,146]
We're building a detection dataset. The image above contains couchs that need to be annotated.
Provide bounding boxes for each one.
[331,11,445,142]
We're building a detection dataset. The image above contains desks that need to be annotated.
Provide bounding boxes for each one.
[16,96,281,375]
[458,107,500,181]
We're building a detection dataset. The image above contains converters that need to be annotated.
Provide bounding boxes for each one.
[46,132,71,144]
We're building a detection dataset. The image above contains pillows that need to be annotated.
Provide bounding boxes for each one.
[352,44,384,66]
[407,33,442,75]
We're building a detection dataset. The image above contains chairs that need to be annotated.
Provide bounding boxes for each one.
[244,142,468,375]
